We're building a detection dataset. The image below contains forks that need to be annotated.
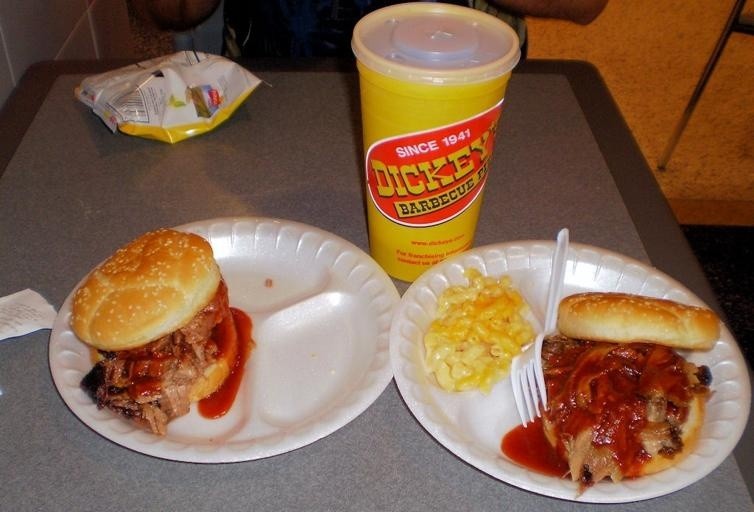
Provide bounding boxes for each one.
[510,228,570,431]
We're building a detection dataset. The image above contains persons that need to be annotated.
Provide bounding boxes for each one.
[128,1,607,72]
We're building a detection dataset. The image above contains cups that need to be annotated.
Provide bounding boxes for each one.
[348,2,525,287]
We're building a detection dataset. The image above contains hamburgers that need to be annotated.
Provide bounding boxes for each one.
[70,228,237,436]
[538,292,720,485]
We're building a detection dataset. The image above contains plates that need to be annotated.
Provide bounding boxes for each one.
[385,241,753,506]
[46,214,403,467]
